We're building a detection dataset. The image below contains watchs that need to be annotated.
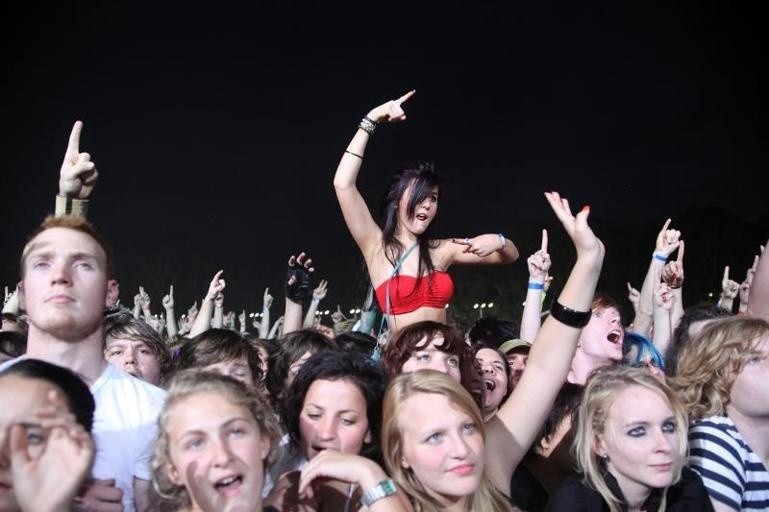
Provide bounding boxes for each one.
[362,478,399,507]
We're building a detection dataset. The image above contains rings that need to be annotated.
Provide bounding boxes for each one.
[465,239,470,244]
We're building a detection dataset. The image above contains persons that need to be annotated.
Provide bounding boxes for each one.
[0,215,170,511]
[677,316,769,512]
[520,228,552,344]
[175,328,263,391]
[542,366,714,512]
[103,321,171,388]
[0,359,125,512]
[470,346,511,425]
[512,382,585,511]
[0,313,28,330]
[151,371,408,512]
[462,346,487,411]
[334,90,519,336]
[666,304,736,378]
[0,331,26,365]
[619,332,665,384]
[497,339,533,391]
[470,315,520,354]
[262,350,389,496]
[266,329,338,407]
[627,218,684,357]
[353,191,605,512]
[380,320,462,383]
[717,240,769,315]
[133,251,343,338]
[332,332,378,359]
[565,291,624,394]
[53,118,99,219]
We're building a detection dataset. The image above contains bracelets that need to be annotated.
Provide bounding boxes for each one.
[358,115,376,135]
[653,252,667,262]
[550,300,592,329]
[54,195,89,224]
[527,283,545,290]
[498,233,505,253]
[343,150,365,160]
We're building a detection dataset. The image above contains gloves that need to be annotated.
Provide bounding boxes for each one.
[283,261,313,303]
